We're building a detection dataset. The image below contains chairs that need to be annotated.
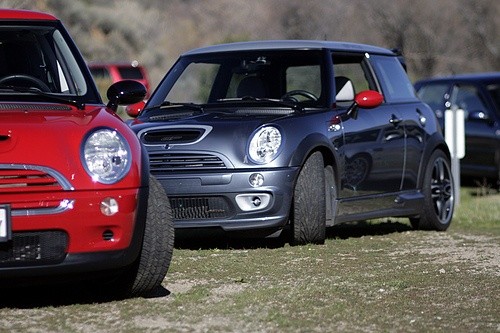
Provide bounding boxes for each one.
[334,76,356,101]
[237,76,271,98]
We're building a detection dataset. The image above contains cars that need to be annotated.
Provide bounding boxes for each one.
[0,8,174,297]
[86,62,152,121]
[413,72,500,180]
[125,39,455,242]
